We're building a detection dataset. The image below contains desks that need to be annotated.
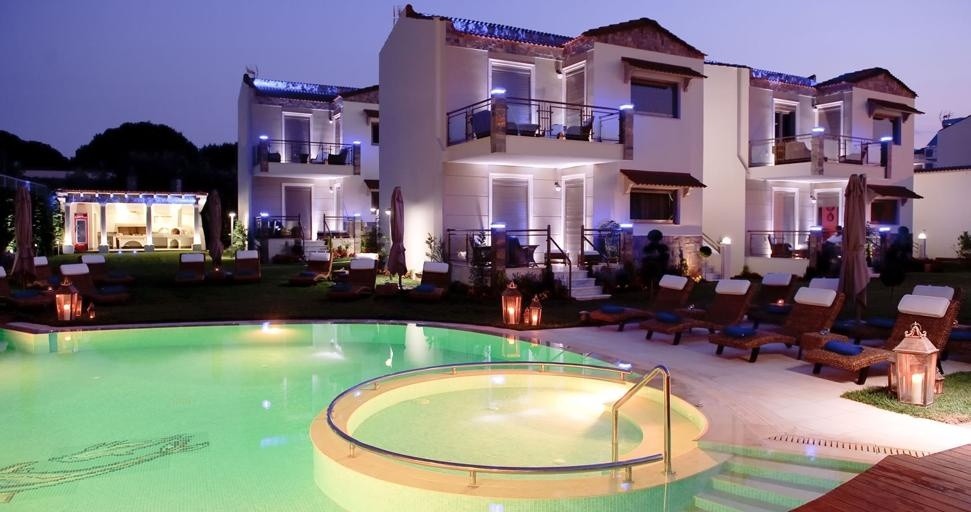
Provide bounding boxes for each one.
[796,332,849,361]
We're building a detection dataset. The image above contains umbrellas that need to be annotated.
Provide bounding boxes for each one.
[836,172,871,346]
[206,186,225,269]
[9,186,37,290]
[386,187,408,289]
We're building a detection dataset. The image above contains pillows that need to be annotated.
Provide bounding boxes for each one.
[600,304,624,315]
[724,324,754,339]
[655,310,680,323]
[825,339,864,357]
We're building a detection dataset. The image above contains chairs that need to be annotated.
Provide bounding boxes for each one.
[801,294,961,381]
[706,286,846,363]
[170,246,451,303]
[638,278,757,346]
[587,274,694,332]
[809,276,844,292]
[744,272,806,329]
[0,248,108,309]
[831,297,899,346]
[470,110,595,143]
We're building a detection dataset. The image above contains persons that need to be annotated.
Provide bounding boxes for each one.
[641,229,669,292]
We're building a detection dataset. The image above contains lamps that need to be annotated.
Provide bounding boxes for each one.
[500,282,542,330]
[892,321,940,406]
